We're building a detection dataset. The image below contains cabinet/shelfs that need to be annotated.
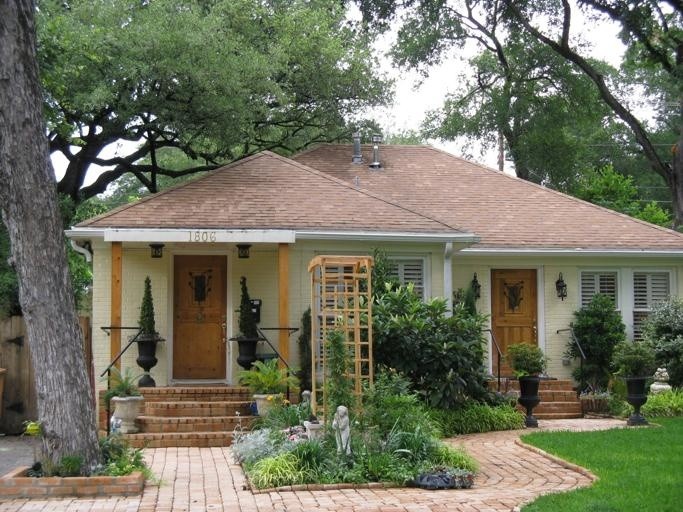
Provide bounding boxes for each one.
[304,254,376,422]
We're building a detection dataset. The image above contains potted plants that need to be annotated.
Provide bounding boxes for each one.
[98,363,147,433]
[227,273,266,386]
[233,356,298,417]
[127,274,166,388]
[506,338,546,428]
[610,342,654,427]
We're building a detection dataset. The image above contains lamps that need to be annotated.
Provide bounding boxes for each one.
[148,244,165,258]
[472,271,481,301]
[555,271,567,301]
[236,243,251,259]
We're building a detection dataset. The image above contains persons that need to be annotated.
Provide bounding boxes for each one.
[332,405,350,455]
